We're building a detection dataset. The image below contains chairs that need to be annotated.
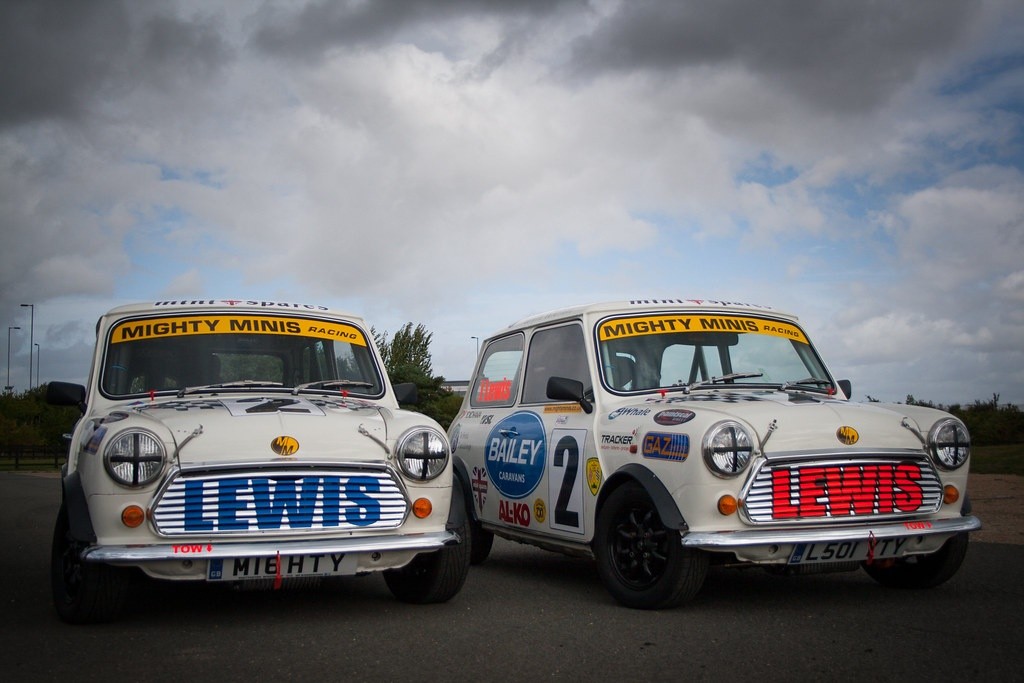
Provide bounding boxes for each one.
[130,365,168,392]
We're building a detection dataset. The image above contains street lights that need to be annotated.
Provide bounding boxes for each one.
[471,337,478,359]
[34,343,39,388]
[5,386,12,394]
[20,303,34,389]
[7,327,20,394]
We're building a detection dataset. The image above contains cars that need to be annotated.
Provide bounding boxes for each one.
[47,295,472,618]
[446,298,981,609]
